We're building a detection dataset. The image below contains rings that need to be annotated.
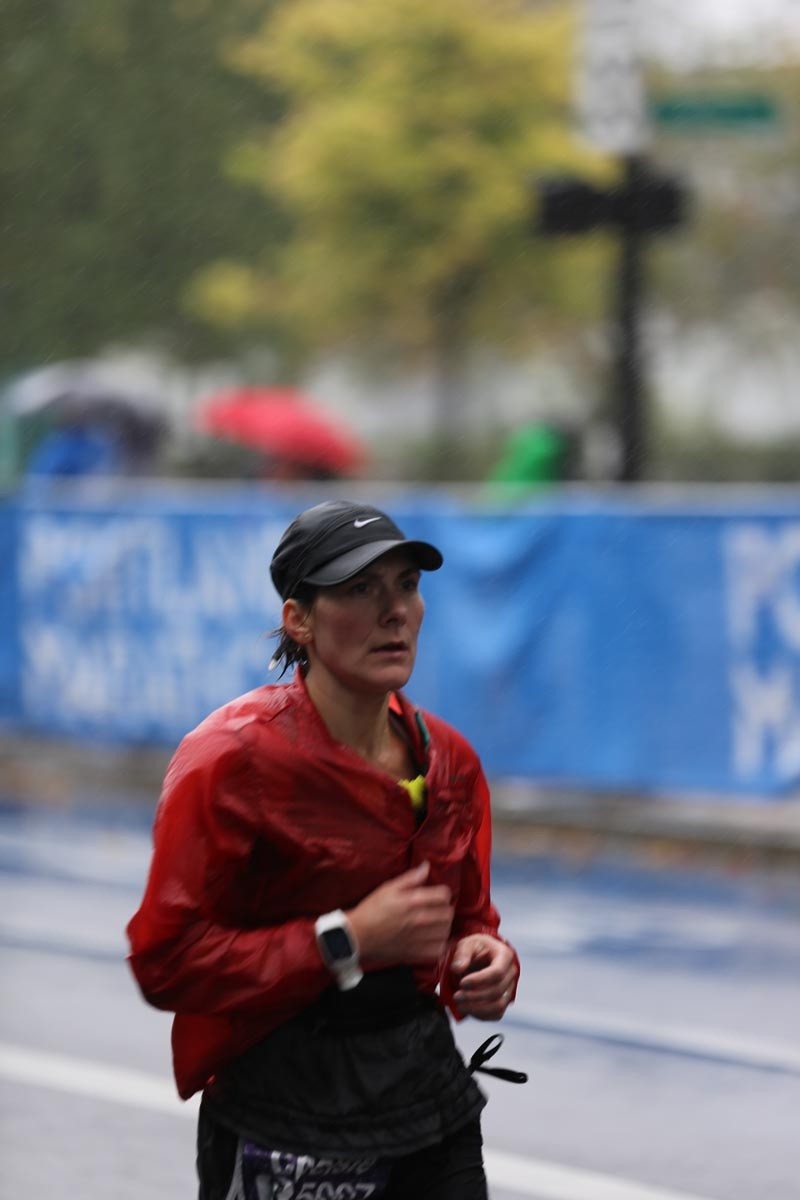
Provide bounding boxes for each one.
[502,991,511,1001]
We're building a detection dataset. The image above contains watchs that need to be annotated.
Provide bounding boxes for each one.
[315,909,362,990]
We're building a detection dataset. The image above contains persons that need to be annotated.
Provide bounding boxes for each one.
[274,455,341,485]
[484,421,562,487]
[27,398,166,483]
[123,499,521,1200]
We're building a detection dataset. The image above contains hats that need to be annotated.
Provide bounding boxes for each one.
[268,498,444,605]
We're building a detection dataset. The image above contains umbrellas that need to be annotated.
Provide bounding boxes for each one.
[5,350,188,424]
[185,378,370,470]
